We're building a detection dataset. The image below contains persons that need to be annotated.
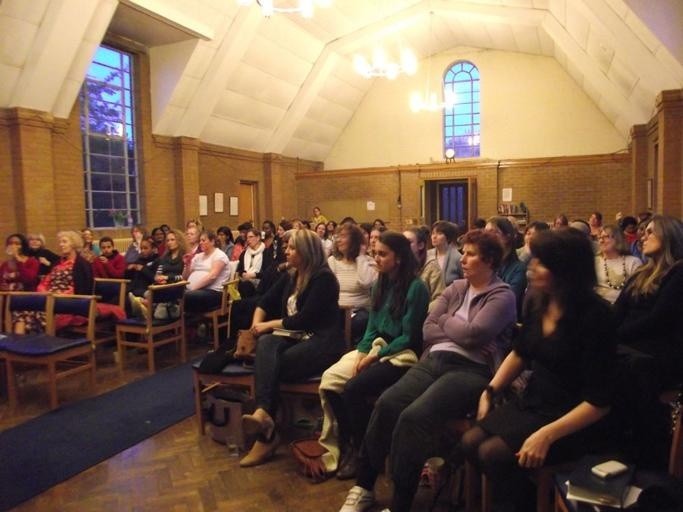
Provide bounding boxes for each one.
[318,231,428,481]
[340,228,516,511]
[475,207,682,511]
[272,208,465,347]
[1,218,283,336]
[241,230,340,467]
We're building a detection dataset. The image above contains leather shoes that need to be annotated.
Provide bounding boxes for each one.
[336,445,375,512]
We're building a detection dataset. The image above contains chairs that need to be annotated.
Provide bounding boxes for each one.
[191,304,683,512]
[0,271,241,410]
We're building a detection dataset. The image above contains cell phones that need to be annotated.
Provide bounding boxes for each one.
[591,460,629,481]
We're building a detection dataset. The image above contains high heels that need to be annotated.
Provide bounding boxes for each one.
[240,432,279,467]
[242,408,275,441]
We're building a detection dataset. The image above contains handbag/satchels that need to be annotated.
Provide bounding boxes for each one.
[289,437,328,483]
[204,389,256,449]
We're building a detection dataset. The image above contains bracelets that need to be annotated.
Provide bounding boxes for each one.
[485,384,495,397]
